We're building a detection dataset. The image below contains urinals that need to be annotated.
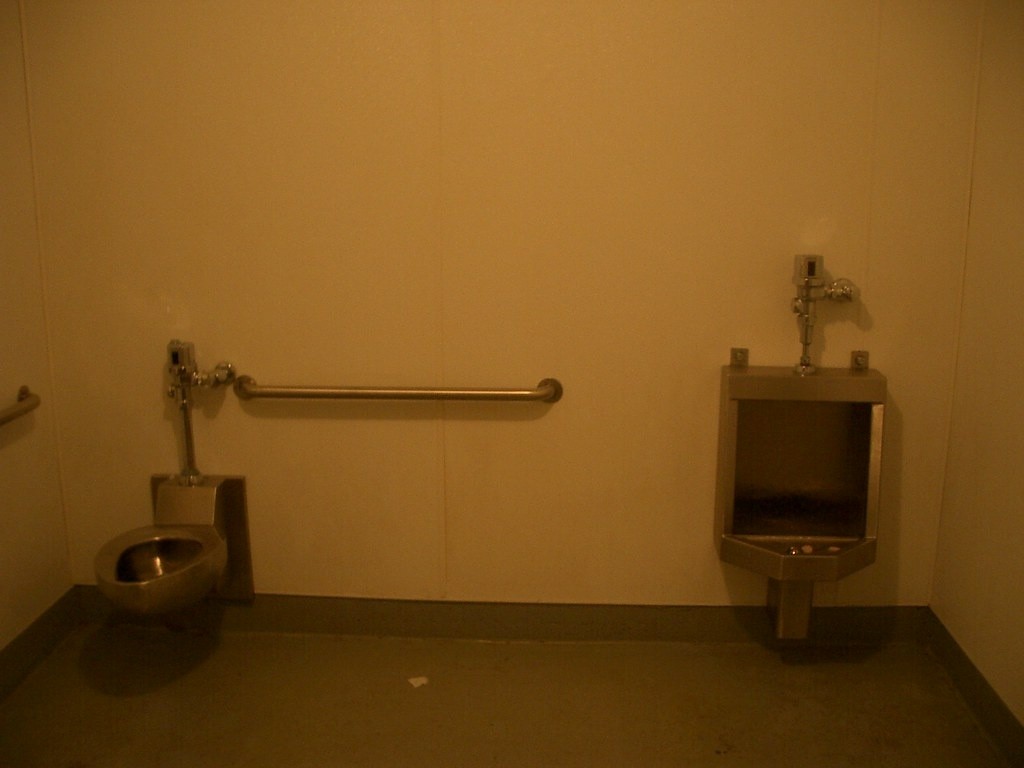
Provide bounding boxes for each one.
[713,346,886,637]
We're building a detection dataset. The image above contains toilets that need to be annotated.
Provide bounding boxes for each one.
[91,472,257,619]
